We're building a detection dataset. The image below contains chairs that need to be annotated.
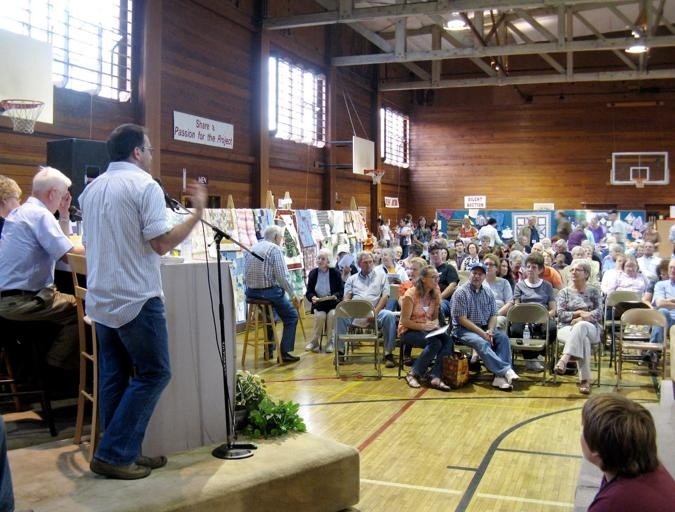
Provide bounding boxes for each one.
[0,254,112,462]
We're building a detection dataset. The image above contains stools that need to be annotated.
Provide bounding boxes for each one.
[241,295,285,370]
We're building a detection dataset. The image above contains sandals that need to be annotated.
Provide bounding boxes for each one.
[430,376,451,392]
[403,372,421,389]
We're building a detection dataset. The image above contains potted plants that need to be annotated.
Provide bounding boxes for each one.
[235,370,266,434]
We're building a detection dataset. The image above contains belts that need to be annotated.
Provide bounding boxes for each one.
[1,289,38,297]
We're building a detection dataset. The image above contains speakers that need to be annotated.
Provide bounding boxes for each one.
[47,139,111,210]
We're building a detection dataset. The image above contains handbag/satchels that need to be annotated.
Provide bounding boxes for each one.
[442,355,471,388]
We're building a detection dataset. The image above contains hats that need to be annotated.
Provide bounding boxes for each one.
[335,243,350,255]
[469,262,488,272]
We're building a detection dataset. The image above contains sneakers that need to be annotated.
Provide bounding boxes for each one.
[526,361,545,373]
[135,455,167,470]
[402,355,412,367]
[303,342,335,354]
[90,457,151,479]
[490,368,521,393]
[261,348,275,361]
[333,350,346,366]
[644,351,660,372]
[382,353,395,368]
[553,359,567,373]
[577,382,593,395]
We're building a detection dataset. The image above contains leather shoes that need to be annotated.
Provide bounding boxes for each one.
[276,351,300,364]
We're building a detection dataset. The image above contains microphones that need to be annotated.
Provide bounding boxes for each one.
[68,205,82,217]
[153,177,174,212]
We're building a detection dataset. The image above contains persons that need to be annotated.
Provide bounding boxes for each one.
[578,391,675,512]
[1,166,87,389]
[240,224,305,366]
[0,175,22,242]
[310,210,675,386]
[79,125,209,480]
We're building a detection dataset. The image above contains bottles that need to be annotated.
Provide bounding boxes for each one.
[523,324,531,347]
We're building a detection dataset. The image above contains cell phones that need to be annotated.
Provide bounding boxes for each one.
[516,339,522,345]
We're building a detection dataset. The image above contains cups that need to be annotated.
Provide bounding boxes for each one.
[170,243,181,256]
[661,380,674,406]
